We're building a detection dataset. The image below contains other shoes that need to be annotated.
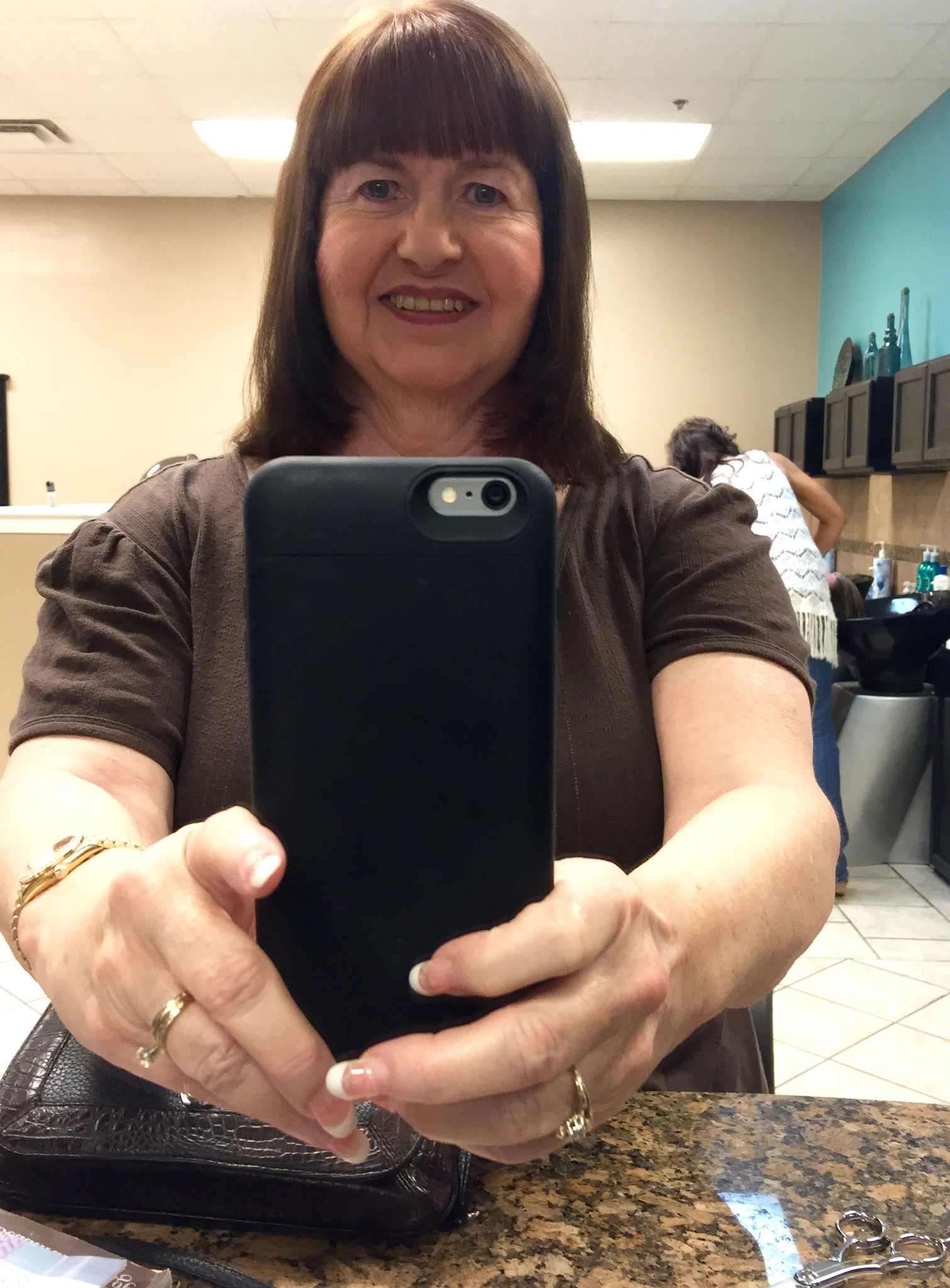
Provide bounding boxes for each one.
[836,880,849,896]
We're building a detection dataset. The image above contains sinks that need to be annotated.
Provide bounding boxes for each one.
[836,591,950,693]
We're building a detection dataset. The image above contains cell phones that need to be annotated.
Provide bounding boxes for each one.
[242,456,558,1062]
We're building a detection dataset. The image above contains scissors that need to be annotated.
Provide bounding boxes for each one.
[792,1208,947,1288]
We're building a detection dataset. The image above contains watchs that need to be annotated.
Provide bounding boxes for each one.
[9,832,147,975]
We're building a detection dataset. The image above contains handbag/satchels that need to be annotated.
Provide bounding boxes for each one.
[0,1001,474,1242]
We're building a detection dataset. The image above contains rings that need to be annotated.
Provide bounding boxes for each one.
[134,990,196,1069]
[557,1065,595,1145]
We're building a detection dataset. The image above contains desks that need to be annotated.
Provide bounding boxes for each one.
[0,1092,948,1288]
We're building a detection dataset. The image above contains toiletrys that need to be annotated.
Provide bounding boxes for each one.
[866,542,893,601]
[901,544,950,593]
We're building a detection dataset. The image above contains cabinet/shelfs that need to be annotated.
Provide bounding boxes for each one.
[821,377,903,476]
[773,396,869,479]
[891,354,950,473]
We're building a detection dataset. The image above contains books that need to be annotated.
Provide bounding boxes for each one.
[0,1207,175,1288]
[0,1231,128,1288]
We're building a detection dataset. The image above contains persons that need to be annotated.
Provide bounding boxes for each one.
[663,415,851,897]
[0,0,845,1173]
[823,572,866,619]
